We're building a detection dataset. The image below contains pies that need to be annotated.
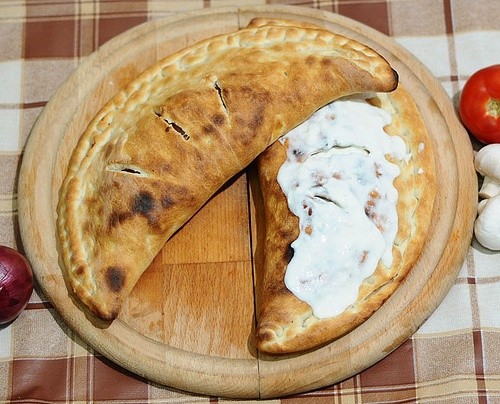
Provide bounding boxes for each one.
[247,17,439,355]
[51,23,400,323]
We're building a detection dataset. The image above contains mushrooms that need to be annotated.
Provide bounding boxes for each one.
[475,143,500,251]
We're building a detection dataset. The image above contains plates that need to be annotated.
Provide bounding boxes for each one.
[15,7,478,397]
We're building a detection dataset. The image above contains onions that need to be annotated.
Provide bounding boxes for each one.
[0,245,34,327]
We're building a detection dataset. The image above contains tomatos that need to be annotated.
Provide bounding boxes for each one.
[458,64,500,144]
